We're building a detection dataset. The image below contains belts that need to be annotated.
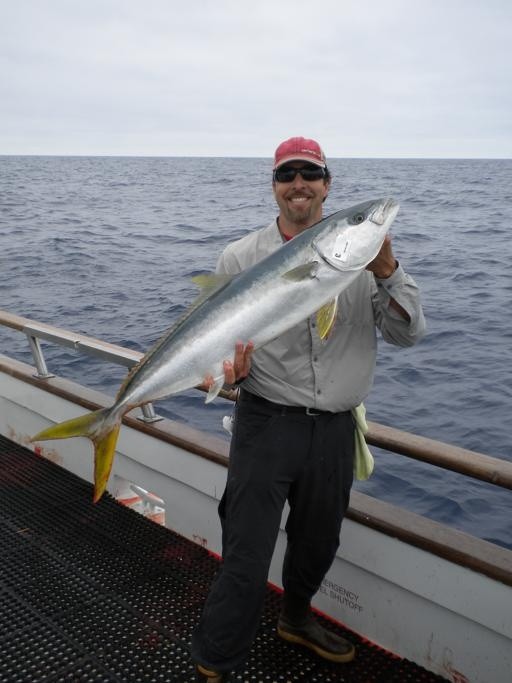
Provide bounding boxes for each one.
[243,392,334,417]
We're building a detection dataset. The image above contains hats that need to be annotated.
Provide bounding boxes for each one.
[273,136,325,173]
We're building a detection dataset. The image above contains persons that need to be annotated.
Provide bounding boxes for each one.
[187,134,427,677]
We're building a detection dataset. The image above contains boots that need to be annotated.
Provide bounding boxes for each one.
[277,604,356,664]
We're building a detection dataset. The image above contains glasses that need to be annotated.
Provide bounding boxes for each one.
[273,169,324,183]
